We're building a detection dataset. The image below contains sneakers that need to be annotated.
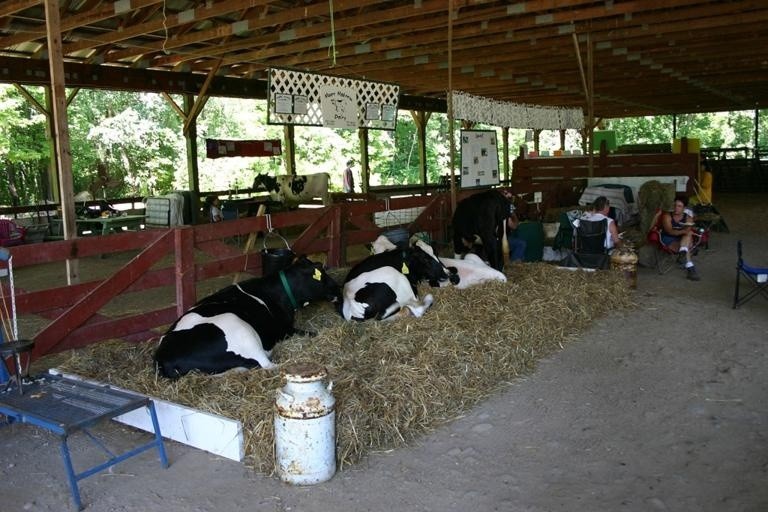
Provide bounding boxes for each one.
[688,272,699,280]
[677,255,687,264]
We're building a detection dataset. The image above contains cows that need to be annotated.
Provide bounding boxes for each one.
[451,189,512,271]
[369,234,508,290]
[339,238,452,325]
[154,253,343,382]
[252,172,332,211]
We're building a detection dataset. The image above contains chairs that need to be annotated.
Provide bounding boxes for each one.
[221,207,240,249]
[0,219,51,246]
[733,240,768,309]
[511,207,710,275]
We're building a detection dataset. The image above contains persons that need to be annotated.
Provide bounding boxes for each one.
[697,159,713,205]
[572,195,624,255]
[450,188,519,273]
[206,195,224,223]
[658,194,696,274]
[343,160,355,193]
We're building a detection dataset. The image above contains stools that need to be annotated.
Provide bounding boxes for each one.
[0,339,40,396]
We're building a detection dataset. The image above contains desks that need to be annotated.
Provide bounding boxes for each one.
[0,372,169,512]
[45,214,150,260]
